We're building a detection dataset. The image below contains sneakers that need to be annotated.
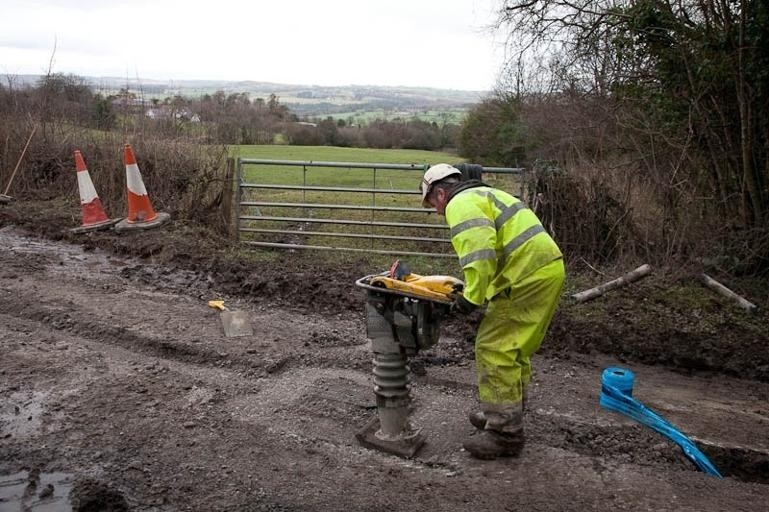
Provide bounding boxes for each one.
[467,408,488,430]
[462,428,526,461]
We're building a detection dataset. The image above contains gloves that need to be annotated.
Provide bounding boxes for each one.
[445,293,478,316]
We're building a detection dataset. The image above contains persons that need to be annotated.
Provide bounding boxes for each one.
[419,162,567,460]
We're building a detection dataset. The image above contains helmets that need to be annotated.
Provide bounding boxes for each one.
[419,161,463,210]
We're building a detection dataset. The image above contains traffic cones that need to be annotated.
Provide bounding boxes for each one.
[71,143,171,234]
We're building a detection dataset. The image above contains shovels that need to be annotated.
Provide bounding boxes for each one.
[208,300,253,337]
